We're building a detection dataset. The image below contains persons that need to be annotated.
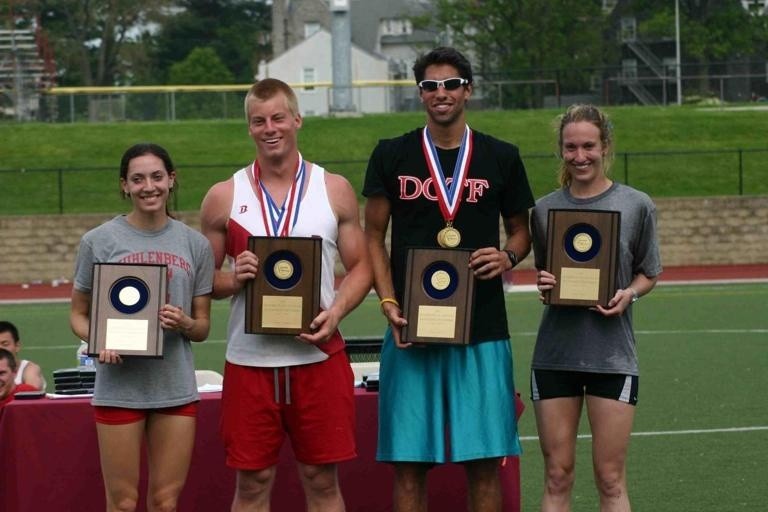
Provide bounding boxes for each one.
[200,78,373,512]
[0,348,38,409]
[533,104,664,512]
[362,46,530,512]
[0,319,47,395]
[71,143,211,512]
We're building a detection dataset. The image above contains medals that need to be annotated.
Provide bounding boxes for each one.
[436,220,462,249]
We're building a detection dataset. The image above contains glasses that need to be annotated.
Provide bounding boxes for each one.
[418,78,470,91]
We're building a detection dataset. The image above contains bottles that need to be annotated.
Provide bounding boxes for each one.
[78,338,93,366]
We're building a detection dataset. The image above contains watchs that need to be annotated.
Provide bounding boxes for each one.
[503,249,518,267]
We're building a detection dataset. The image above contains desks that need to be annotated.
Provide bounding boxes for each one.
[1,364,525,511]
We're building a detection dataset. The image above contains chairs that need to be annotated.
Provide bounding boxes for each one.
[348,360,381,384]
[194,368,224,389]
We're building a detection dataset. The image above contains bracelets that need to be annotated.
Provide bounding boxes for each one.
[630,287,639,304]
[374,297,401,314]
